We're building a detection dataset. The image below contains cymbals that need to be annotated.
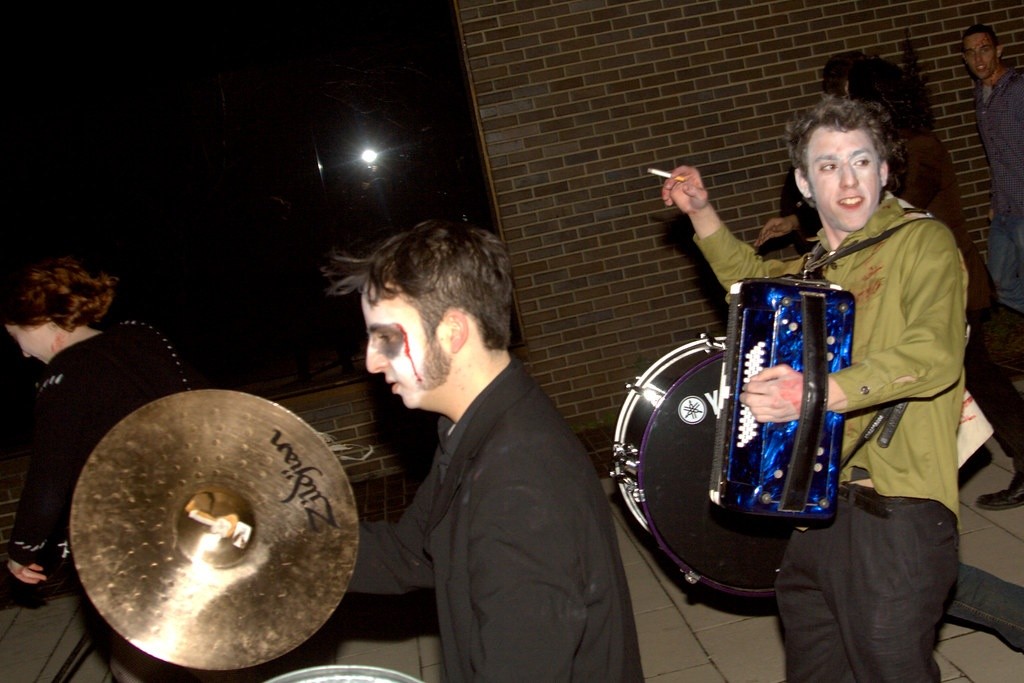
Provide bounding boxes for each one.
[67,387,361,672]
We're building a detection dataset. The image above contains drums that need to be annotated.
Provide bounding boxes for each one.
[609,331,794,600]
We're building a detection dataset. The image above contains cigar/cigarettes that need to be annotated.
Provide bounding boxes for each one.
[648,166,686,183]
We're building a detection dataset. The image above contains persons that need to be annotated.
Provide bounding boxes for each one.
[340,216,651,682]
[959,22,1024,317]
[659,95,973,683]
[1,246,259,683]
[753,48,1024,512]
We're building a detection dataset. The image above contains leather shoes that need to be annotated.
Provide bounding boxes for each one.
[977,470,1024,510]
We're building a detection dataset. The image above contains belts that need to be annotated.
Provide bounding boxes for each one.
[835,479,928,519]
